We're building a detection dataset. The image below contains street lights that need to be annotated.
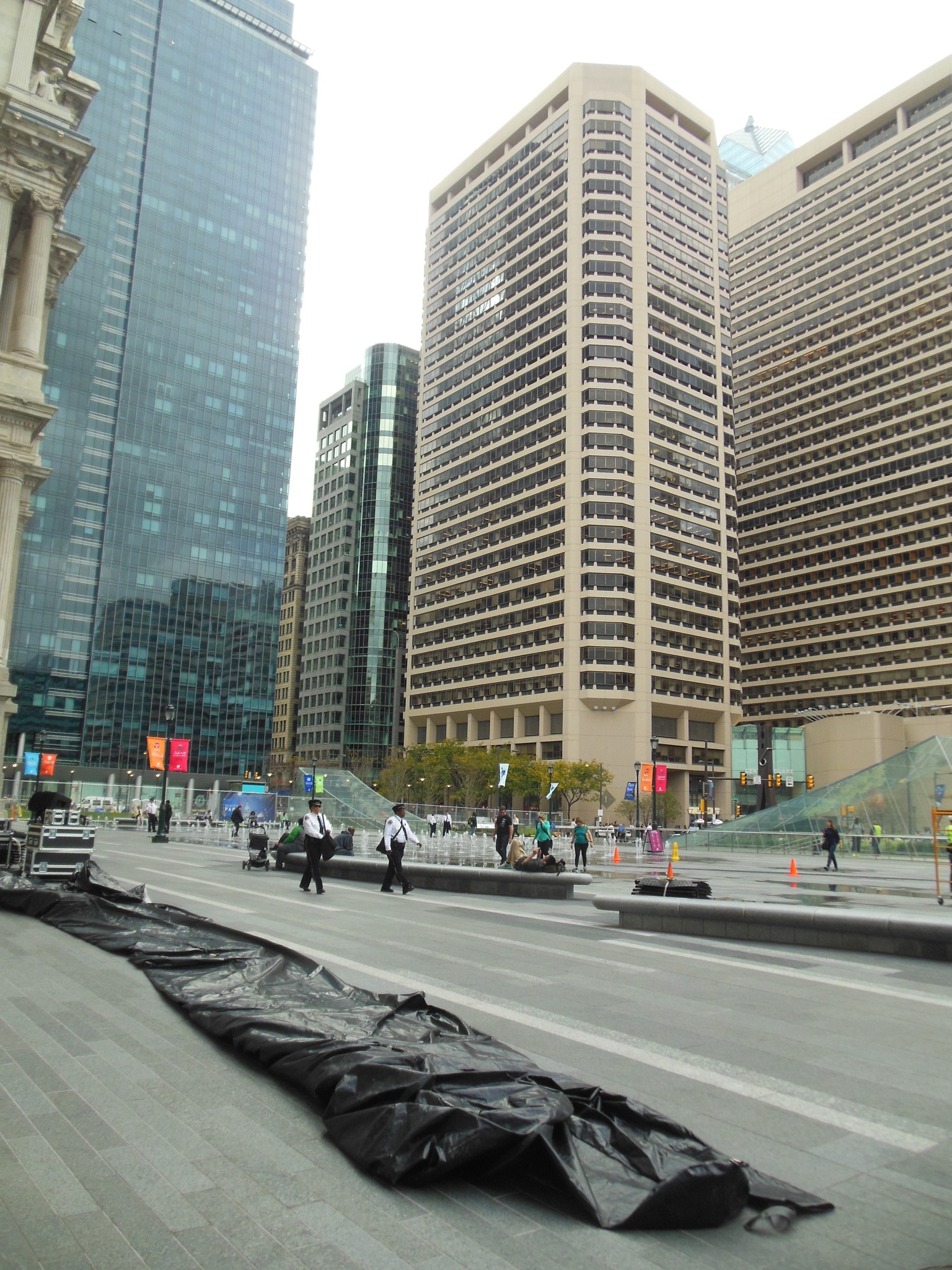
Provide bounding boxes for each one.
[650,736,659,831]
[151,704,177,844]
[68,770,74,798]
[488,785,495,823]
[406,777,452,817]
[266,773,274,793]
[26,730,47,825]
[312,757,318,799]
[634,760,641,844]
[585,763,602,827]
[125,750,160,812]
[13,763,19,778]
[547,764,554,825]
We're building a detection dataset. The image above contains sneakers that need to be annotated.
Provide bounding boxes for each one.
[498,861,512,869]
[572,866,587,871]
[522,839,529,850]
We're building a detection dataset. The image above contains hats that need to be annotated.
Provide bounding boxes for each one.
[392,804,405,811]
[308,799,322,806]
[445,811,449,813]
[150,798,154,800]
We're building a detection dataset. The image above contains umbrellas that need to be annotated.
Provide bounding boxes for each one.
[711,818,723,825]
[692,818,705,824]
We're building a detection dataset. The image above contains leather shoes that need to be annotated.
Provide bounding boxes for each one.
[317,888,325,894]
[381,886,394,892]
[299,884,310,891]
[403,886,414,894]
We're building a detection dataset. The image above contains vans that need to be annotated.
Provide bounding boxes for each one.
[75,796,113,811]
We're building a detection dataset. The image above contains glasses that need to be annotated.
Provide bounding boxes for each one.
[401,809,405,812]
[315,805,321,808]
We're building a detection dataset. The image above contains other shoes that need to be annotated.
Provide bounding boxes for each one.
[269,845,278,850]
[273,866,282,871]
[149,829,157,832]
[825,867,839,872]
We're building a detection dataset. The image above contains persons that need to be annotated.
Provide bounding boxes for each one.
[298,799,331,894]
[133,796,722,871]
[570,817,593,872]
[821,819,841,872]
[846,817,865,855]
[380,805,421,895]
[868,821,883,857]
[533,813,551,859]
[945,815,952,872]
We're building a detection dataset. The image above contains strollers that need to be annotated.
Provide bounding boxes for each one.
[242,825,270,871]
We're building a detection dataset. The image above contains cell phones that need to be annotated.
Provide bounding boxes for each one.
[538,848,540,853]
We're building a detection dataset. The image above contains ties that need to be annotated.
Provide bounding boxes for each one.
[446,815,447,821]
[401,821,408,841]
[317,815,323,834]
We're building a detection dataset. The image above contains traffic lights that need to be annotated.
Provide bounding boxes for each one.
[806,774,814,789]
[255,772,258,780]
[775,773,782,787]
[740,771,746,786]
[736,805,741,817]
[244,770,249,779]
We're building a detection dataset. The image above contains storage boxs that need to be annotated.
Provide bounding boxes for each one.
[24,823,98,853]
[21,848,95,877]
[44,808,65,825]
[64,809,81,826]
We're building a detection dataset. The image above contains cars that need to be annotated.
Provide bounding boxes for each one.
[594,826,631,843]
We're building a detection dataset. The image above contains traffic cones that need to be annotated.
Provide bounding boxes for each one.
[612,846,622,861]
[787,858,800,876]
[666,862,674,879]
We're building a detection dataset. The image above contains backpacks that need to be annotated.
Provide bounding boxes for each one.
[541,858,565,873]
[468,816,475,825]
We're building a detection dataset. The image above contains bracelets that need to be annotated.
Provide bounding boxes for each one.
[590,841,593,842]
[571,842,573,843]
[509,837,512,838]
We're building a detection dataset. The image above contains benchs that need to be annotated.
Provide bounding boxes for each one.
[241,820,283,829]
[112,817,227,828]
[269,848,594,901]
[590,890,952,971]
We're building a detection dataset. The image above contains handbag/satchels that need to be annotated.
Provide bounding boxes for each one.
[822,842,828,850]
[321,833,337,861]
[585,825,591,842]
[376,836,394,854]
[549,839,553,849]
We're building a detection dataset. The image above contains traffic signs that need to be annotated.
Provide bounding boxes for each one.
[786,777,794,787]
